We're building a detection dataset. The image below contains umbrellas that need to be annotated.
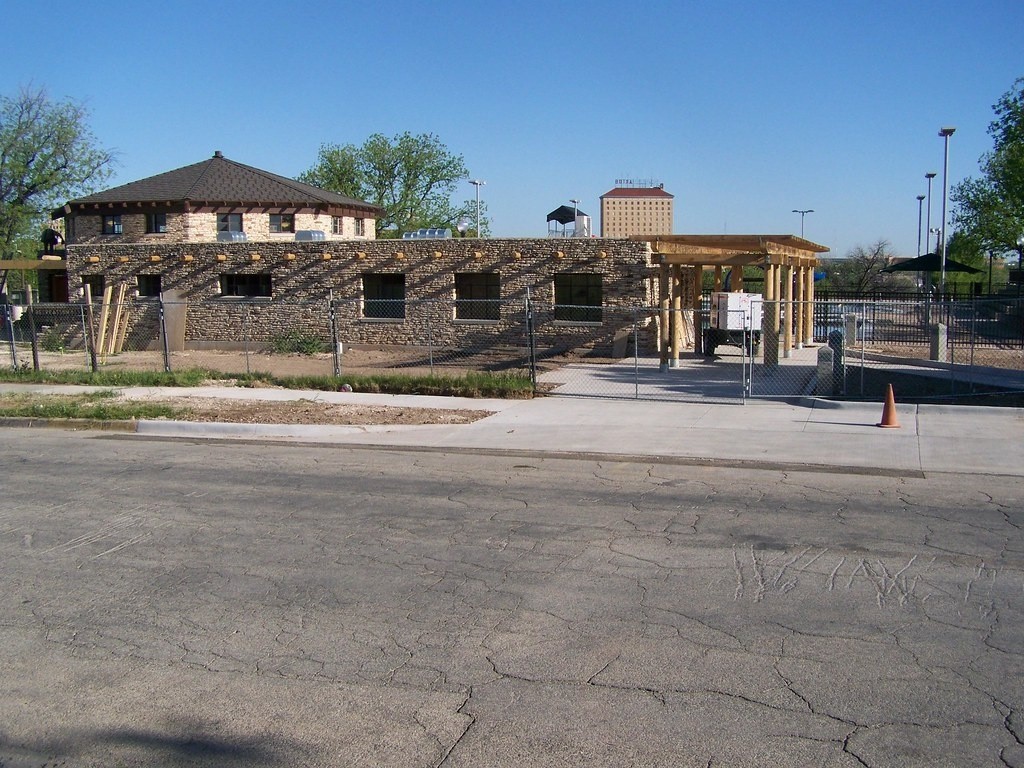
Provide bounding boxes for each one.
[877,252,984,332]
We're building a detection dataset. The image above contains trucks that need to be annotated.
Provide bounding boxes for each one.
[704,290,763,355]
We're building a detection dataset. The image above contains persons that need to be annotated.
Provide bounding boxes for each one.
[41,228,65,255]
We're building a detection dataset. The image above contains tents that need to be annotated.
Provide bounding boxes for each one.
[546,206,589,238]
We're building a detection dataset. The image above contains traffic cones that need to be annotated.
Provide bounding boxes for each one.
[875,383,901,428]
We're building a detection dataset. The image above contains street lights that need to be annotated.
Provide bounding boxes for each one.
[937,127,960,323]
[925,171,937,253]
[915,195,925,304]
[468,179,486,239]
[793,208,814,240]
[570,199,580,234]
[930,227,941,254]
